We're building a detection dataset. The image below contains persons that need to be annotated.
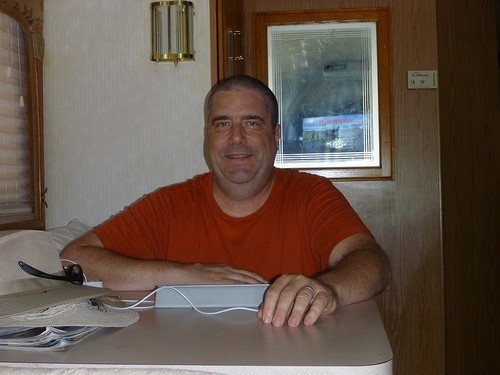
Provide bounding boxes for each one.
[60,74,393,327]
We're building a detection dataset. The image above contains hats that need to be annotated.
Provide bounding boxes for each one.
[0,229,113,319]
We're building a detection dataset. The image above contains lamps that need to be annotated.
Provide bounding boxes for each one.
[150,0,195,64]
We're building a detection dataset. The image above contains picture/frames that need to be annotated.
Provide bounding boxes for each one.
[251,7,396,183]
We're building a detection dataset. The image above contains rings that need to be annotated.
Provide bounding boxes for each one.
[304,286,315,300]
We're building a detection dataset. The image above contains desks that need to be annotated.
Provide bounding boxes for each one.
[0,290,393,375]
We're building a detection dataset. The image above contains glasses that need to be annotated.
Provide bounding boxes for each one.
[18,261,83,285]
